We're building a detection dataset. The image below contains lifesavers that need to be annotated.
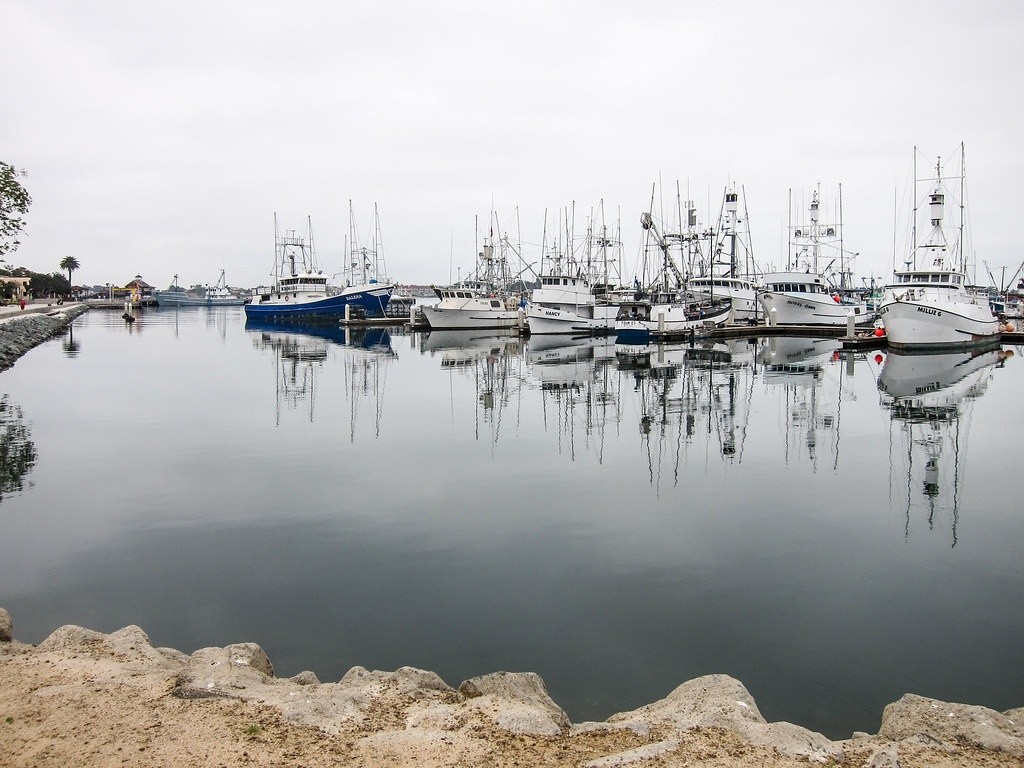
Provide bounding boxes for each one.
[285,296,289,301]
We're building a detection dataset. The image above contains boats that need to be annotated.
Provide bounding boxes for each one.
[420,329,1002,546]
[244,319,399,443]
[422,139,1016,351]
[244,211,396,318]
[140,295,157,307]
[152,272,244,307]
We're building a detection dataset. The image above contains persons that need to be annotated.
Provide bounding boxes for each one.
[20,299,26,310]
